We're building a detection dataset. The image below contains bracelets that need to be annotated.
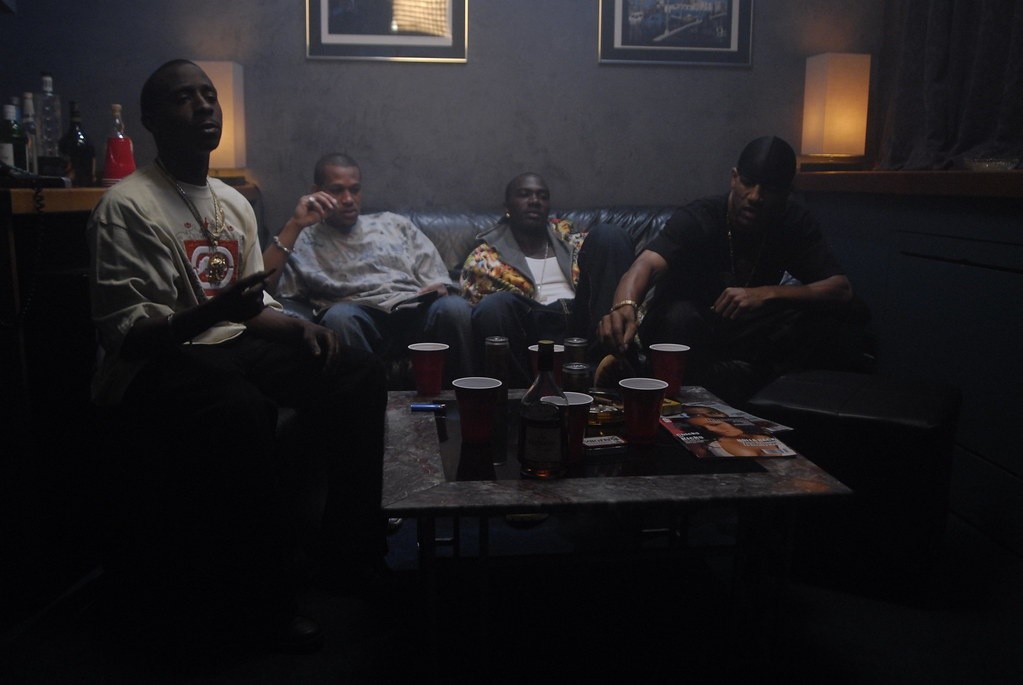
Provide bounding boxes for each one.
[167,312,193,349]
[273,236,296,255]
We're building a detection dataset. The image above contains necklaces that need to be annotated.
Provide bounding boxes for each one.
[725,212,767,287]
[154,154,228,285]
[544,241,549,259]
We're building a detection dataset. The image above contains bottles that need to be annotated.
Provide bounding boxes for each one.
[0,72,126,183]
[518,340,572,462]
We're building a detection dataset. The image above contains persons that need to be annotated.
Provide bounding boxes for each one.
[673,416,796,457]
[259,153,462,393]
[683,402,742,418]
[458,170,638,391]
[598,134,857,400]
[81,60,406,654]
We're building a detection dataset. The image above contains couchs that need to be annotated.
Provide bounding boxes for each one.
[269,205,677,563]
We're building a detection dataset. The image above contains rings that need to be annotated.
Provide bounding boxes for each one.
[308,196,314,206]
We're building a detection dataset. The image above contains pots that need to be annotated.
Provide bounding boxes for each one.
[963,159,1020,171]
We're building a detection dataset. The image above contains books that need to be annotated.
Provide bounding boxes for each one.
[657,399,797,461]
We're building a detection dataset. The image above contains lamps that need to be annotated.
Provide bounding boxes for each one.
[189,59,249,187]
[800,52,870,172]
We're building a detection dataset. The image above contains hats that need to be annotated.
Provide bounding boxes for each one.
[739,137,797,185]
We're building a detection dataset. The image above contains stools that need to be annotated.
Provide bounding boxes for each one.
[746,369,962,613]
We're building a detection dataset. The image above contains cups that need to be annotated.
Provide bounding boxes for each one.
[452,377,502,441]
[527,345,565,397]
[619,378,668,441]
[409,343,450,396]
[650,343,690,397]
[101,137,136,187]
[539,391,594,453]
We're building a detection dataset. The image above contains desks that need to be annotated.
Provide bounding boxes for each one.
[380,385,851,685]
[12,178,261,281]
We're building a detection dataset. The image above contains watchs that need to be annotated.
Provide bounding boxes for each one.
[608,300,649,328]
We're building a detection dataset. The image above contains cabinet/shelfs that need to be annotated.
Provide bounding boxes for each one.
[794,170,1023,563]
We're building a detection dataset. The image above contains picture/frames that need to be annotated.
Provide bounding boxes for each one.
[598,0,756,69]
[305,0,469,65]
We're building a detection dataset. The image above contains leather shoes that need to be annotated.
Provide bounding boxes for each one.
[269,612,323,654]
